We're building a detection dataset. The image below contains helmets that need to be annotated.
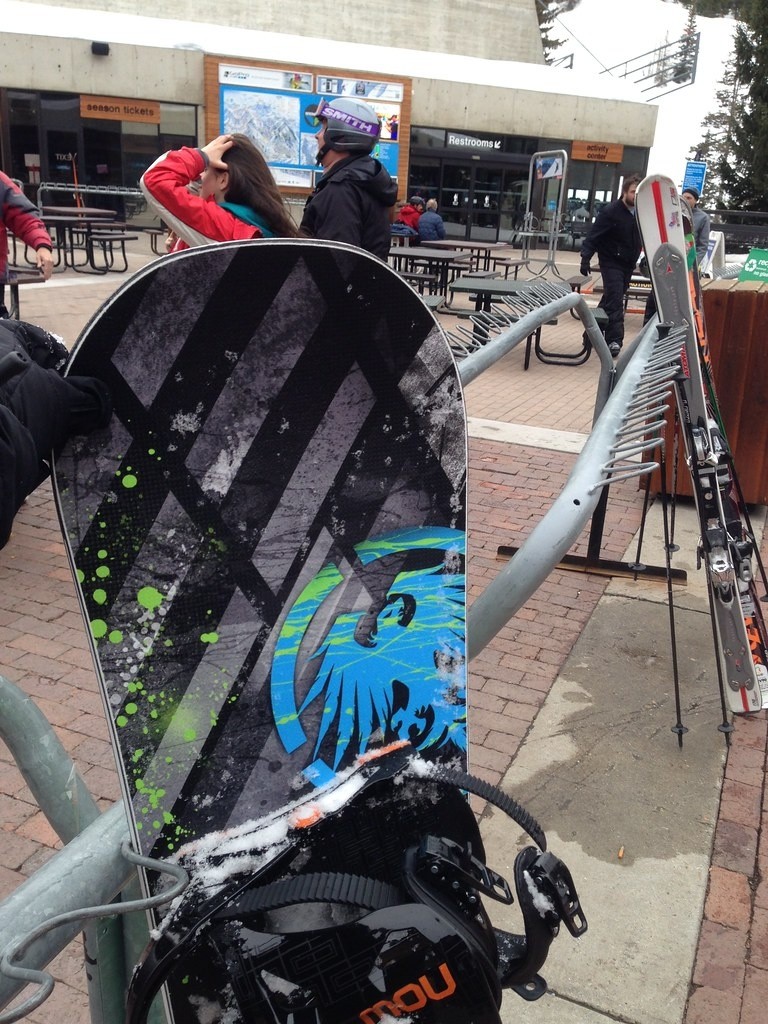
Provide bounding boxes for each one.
[317,97,381,152]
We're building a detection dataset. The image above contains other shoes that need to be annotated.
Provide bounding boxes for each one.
[608,342,620,355]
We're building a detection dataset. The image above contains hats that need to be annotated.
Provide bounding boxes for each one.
[410,196,426,204]
[682,186,700,201]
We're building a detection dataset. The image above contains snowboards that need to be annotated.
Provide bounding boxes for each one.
[37,231,495,1024]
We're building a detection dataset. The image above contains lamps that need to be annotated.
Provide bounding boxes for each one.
[92,42,110,55]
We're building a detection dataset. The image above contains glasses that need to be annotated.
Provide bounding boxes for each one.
[314,98,381,136]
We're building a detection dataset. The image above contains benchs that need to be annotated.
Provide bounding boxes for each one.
[568,276,593,293]
[420,295,446,314]
[463,270,501,278]
[469,294,555,305]
[414,260,470,272]
[14,223,162,275]
[593,285,651,316]
[494,258,532,281]
[455,309,559,370]
[630,278,652,286]
[396,271,434,294]
[411,262,469,281]
[473,254,512,261]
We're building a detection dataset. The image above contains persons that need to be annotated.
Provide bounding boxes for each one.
[397,195,445,246]
[295,98,398,262]
[0,319,113,554]
[380,113,400,143]
[580,174,644,355]
[138,133,307,254]
[638,188,709,325]
[0,169,54,320]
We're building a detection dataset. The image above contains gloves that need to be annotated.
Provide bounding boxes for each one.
[580,258,591,276]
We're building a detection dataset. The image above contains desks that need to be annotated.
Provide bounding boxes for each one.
[40,217,116,272]
[387,246,472,306]
[448,276,572,360]
[590,262,642,276]
[421,238,507,271]
[44,206,121,248]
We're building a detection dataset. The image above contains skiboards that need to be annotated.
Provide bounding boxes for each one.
[628,175,768,733]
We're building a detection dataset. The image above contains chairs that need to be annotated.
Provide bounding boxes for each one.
[578,308,608,368]
